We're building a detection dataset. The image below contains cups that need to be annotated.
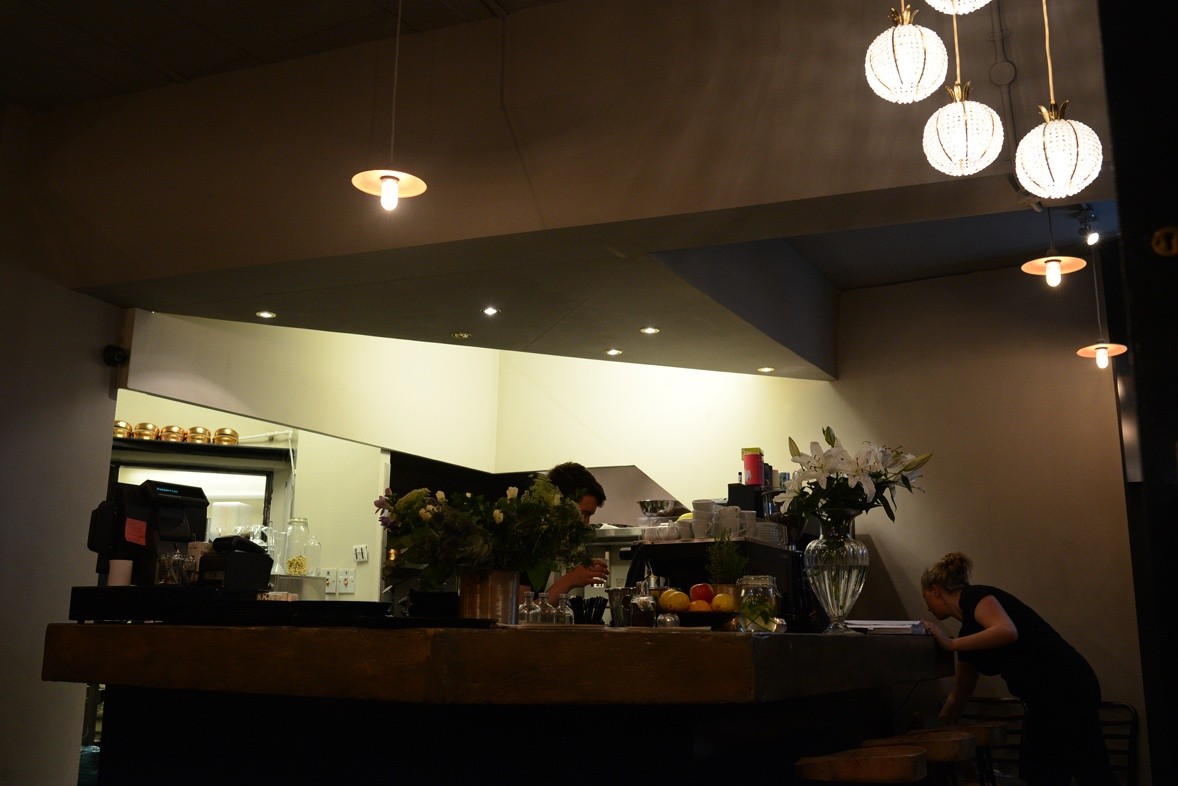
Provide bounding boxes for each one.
[644,499,756,538]
[592,559,608,589]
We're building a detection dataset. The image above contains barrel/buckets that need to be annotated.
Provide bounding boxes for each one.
[606,587,639,626]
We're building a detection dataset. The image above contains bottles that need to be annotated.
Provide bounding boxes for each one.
[631,582,657,627]
[277,516,323,577]
[517,591,574,625]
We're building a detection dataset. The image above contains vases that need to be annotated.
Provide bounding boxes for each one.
[804,509,871,635]
[460,564,523,627]
[407,584,467,618]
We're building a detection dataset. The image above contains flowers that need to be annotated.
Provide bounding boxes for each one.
[374,484,474,593]
[770,425,933,602]
[464,473,596,595]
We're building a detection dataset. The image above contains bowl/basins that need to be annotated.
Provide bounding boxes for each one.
[638,498,679,517]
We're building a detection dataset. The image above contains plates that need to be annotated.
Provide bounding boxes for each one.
[755,521,780,543]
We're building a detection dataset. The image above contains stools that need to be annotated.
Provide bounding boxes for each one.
[861,729,977,785]
[792,743,929,786]
[912,716,1009,786]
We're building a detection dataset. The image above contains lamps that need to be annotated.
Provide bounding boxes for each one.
[1069,203,1100,246]
[349,0,429,213]
[864,0,948,104]
[921,0,1006,178]
[1075,245,1128,370]
[1014,0,1104,200]
[1021,205,1089,286]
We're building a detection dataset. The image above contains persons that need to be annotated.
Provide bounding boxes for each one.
[919,552,1100,786]
[519,461,612,616]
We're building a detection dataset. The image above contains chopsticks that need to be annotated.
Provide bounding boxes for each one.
[568,594,609,624]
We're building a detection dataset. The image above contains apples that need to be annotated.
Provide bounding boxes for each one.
[690,583,714,604]
[712,593,735,612]
[659,589,690,611]
[689,600,711,612]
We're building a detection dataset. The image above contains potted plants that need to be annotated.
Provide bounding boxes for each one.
[700,527,758,632]
